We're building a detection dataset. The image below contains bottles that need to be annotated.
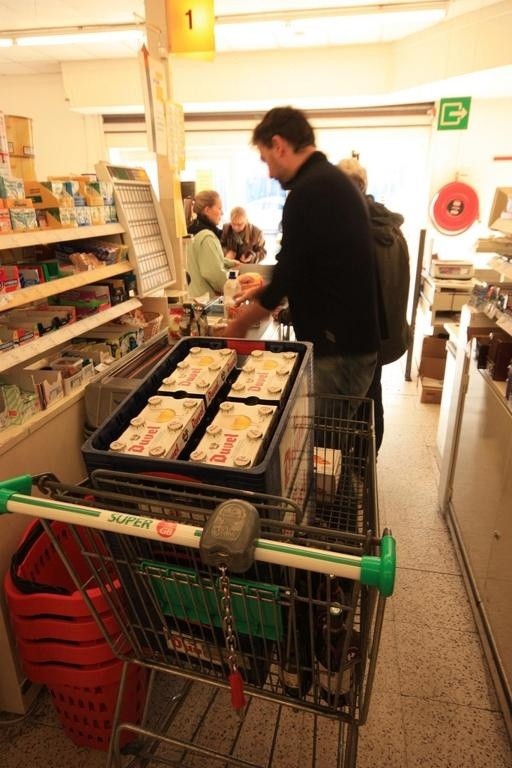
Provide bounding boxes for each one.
[223,271,242,320]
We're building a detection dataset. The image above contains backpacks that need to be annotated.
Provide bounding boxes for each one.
[345,172,410,366]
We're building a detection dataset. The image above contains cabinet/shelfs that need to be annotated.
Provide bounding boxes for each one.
[0,220,173,716]
[433,188,512,746]
[413,270,482,404]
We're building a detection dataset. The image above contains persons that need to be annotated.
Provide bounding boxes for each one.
[219,206,269,264]
[330,154,413,482]
[225,104,389,599]
[182,188,231,304]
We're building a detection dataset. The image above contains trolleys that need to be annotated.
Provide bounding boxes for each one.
[2,396,397,766]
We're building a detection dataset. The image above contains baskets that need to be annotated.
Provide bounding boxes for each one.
[5,472,201,752]
